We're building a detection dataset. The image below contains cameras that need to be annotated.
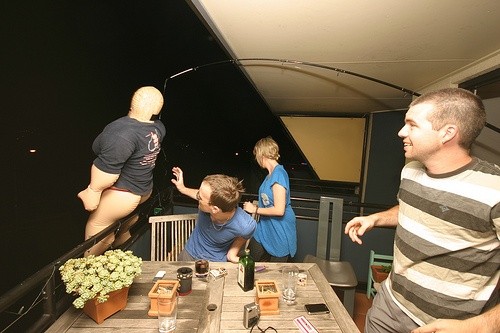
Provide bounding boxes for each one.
[243,302,260,329]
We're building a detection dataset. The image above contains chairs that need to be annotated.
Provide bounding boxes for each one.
[148,214,199,261]
[366,249,394,299]
[303,196,358,319]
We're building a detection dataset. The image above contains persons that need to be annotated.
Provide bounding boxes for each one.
[243,137,297,262]
[77,86,165,256]
[171,167,257,263]
[344,88,500,333]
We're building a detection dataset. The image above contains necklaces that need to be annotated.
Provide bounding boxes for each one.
[211,216,230,232]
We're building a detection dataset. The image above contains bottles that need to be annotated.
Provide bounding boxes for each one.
[238,248,255,292]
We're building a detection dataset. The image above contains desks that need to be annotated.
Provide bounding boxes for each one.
[44,263,362,333]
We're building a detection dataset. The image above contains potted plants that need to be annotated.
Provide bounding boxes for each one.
[371,265,391,283]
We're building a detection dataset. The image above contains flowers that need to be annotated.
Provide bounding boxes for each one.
[59,248,142,308]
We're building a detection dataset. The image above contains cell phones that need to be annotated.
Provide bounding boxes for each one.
[304,303,329,315]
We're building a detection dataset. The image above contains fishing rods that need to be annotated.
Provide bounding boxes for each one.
[164,58,500,132]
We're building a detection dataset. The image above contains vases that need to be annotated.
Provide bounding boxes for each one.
[77,286,128,325]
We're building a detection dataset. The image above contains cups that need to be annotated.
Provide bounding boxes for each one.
[157,290,178,333]
[195,260,209,278]
[177,267,193,296]
[282,265,299,305]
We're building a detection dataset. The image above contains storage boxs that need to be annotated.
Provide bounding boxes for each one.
[255,281,282,315]
[148,279,179,316]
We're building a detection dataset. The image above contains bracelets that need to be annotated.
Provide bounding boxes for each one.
[255,206,258,213]
